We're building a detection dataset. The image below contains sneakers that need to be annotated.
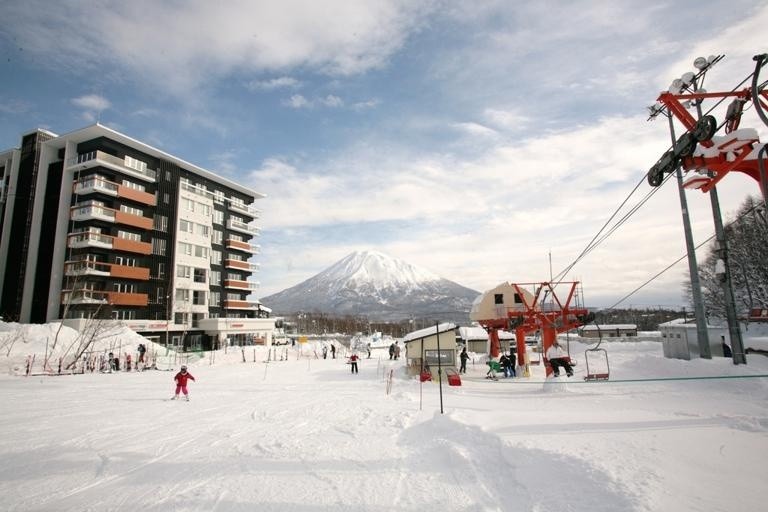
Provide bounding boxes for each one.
[186,397,189,400]
[172,397,179,399]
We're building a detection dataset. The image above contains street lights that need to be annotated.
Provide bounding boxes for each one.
[166,295,170,346]
[646,54,713,361]
[184,297,188,345]
[673,47,748,366]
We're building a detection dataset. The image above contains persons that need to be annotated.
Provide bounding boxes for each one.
[420,365,432,382]
[486,351,516,376]
[323,344,335,359]
[175,364,196,397]
[389,341,400,360]
[350,354,360,374]
[546,341,572,377]
[366,343,371,358]
[460,347,471,373]
[110,344,146,371]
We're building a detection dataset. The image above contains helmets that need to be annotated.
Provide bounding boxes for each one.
[181,366,187,375]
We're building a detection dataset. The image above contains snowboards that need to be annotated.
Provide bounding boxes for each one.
[163,395,190,402]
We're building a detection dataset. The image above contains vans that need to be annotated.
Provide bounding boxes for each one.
[272,334,290,345]
